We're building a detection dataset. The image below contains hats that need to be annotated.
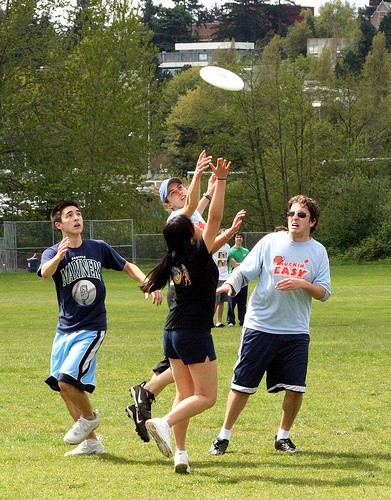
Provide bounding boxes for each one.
[159,178,182,202]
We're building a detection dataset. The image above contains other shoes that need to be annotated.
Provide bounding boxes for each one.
[228,323,233,327]
[216,323,225,327]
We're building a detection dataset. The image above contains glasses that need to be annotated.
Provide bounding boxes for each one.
[287,211,310,218]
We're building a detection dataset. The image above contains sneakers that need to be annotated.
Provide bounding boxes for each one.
[129,381,155,420]
[174,451,190,474]
[144,417,173,458]
[274,434,303,453]
[209,436,229,455]
[125,404,151,442]
[64,436,104,457]
[64,409,99,445]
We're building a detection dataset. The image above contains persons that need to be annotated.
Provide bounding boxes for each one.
[211,195,331,457]
[37,200,162,457]
[125,151,216,443]
[210,228,230,327]
[274,227,289,232]
[227,232,250,326]
[145,157,245,474]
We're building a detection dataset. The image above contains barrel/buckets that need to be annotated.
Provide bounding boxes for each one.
[27,258,37,273]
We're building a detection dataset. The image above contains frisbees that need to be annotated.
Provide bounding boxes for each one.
[199,66,245,92]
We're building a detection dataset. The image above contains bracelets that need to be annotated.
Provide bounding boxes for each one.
[203,193,212,202]
[216,178,227,181]
